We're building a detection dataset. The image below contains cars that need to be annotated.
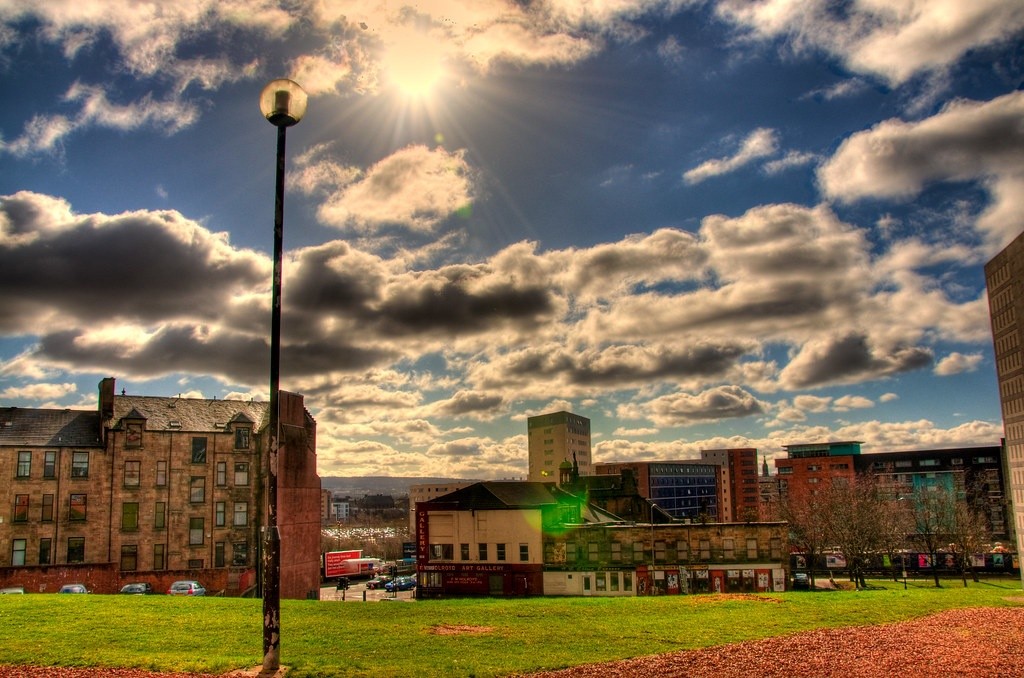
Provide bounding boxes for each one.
[165,581,207,597]
[58,584,88,595]
[366,574,395,590]
[0,587,26,596]
[793,573,810,592]
[384,577,416,592]
[120,582,152,594]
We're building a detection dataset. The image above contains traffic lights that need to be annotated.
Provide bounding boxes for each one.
[336,577,351,590]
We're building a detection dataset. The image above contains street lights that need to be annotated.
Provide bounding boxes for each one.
[258,74,308,667]
[650,503,658,596]
